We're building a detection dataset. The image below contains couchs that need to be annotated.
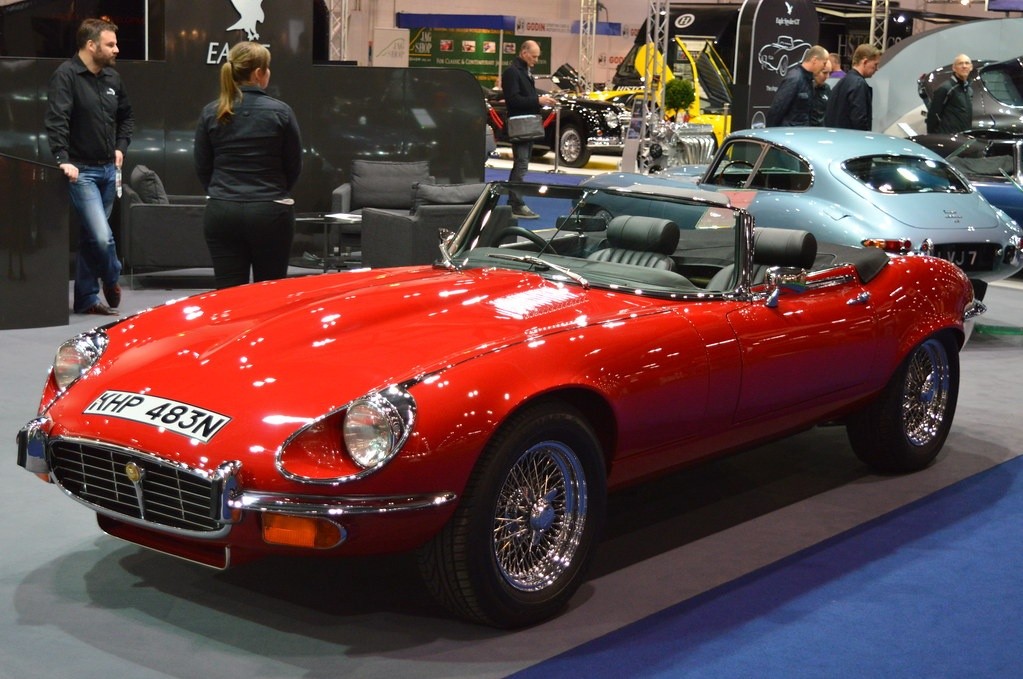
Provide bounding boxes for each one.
[360,204,517,271]
[121,184,216,288]
[331,176,451,247]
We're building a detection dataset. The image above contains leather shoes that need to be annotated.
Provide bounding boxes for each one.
[103,282,123,308]
[84,302,120,316]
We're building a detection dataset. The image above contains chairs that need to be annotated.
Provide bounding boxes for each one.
[705,226,817,291]
[586,214,681,273]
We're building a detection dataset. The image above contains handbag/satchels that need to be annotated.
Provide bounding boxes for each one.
[507,114,545,143]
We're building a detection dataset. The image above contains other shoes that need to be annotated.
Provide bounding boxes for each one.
[511,205,540,219]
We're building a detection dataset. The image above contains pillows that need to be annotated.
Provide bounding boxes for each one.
[351,159,430,207]
[129,164,170,205]
[408,182,487,216]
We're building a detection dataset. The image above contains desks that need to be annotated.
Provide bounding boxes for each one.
[295,211,361,274]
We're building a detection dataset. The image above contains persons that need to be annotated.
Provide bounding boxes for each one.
[193,42,303,290]
[828,53,846,79]
[45,19,136,316]
[809,60,832,126]
[825,44,880,132]
[766,45,829,128]
[926,54,973,134]
[502,40,557,218]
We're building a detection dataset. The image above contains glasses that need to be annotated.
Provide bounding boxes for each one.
[822,70,833,76]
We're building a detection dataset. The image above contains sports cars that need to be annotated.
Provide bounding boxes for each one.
[573,127,1023,304]
[17,186,976,629]
[479,37,663,169]
